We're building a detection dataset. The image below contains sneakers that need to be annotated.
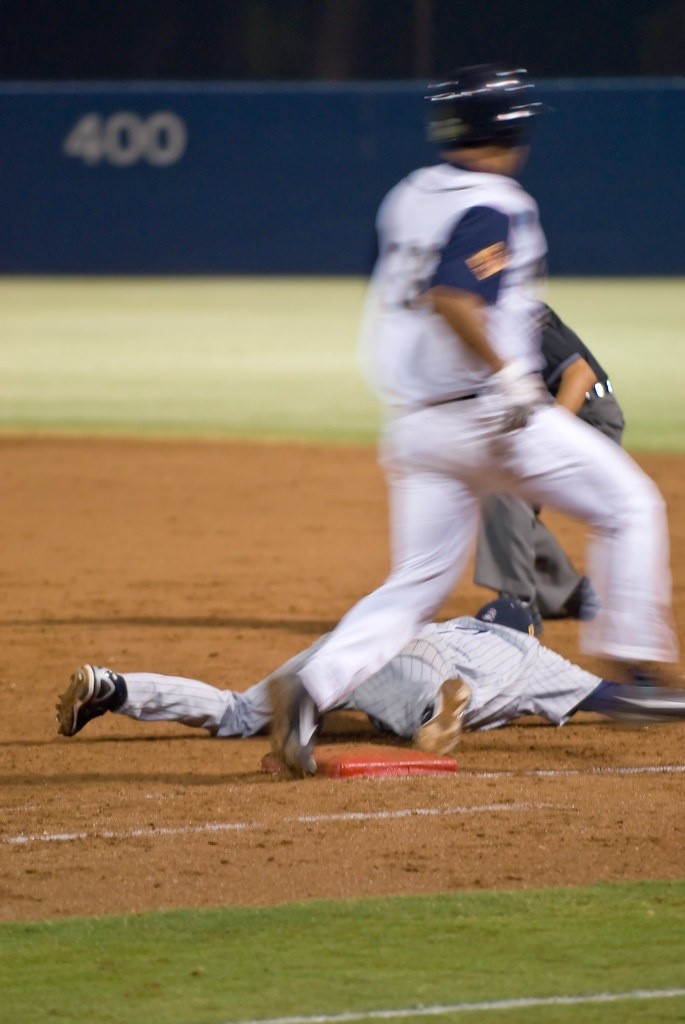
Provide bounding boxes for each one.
[56,663,127,737]
[268,674,320,778]
[415,679,471,756]
[608,685,685,722]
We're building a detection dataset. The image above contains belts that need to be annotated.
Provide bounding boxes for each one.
[432,393,477,406]
[586,380,613,399]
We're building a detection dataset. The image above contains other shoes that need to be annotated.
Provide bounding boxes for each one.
[540,576,602,622]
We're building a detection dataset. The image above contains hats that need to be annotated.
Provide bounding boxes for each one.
[475,599,536,636]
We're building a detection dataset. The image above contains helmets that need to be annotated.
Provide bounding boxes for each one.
[424,61,541,148]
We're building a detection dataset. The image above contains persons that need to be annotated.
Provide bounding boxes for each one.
[53,296,624,756]
[265,61,685,778]
